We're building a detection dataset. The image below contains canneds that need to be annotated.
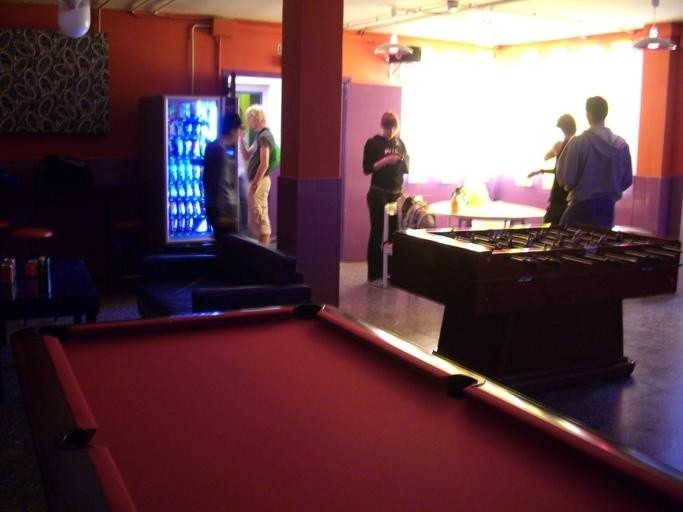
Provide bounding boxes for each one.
[6,286,16,300]
[1,256,16,285]
[170,201,206,232]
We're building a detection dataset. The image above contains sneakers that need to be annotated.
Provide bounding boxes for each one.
[367,279,384,289]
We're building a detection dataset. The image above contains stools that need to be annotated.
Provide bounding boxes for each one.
[0,217,145,297]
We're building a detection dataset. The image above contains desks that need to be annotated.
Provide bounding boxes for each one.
[428,198,547,228]
[0,258,99,347]
[11,303,683,512]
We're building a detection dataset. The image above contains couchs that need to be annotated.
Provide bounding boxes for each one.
[137,234,312,318]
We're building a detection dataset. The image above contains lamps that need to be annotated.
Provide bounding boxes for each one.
[633,0,678,50]
[374,5,414,57]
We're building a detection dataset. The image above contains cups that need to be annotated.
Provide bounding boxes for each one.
[169,100,209,238]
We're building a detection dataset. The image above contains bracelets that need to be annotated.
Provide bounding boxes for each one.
[541,169,544,175]
[252,181,257,189]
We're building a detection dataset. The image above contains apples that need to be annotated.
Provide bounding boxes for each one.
[24,259,39,276]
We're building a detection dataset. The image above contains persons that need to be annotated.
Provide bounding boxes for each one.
[526,114,576,224]
[203,112,243,259]
[362,113,409,282]
[238,105,274,247]
[556,97,632,225]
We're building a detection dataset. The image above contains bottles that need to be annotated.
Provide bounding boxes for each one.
[3,284,53,303]
[0,255,53,283]
[451,195,459,213]
